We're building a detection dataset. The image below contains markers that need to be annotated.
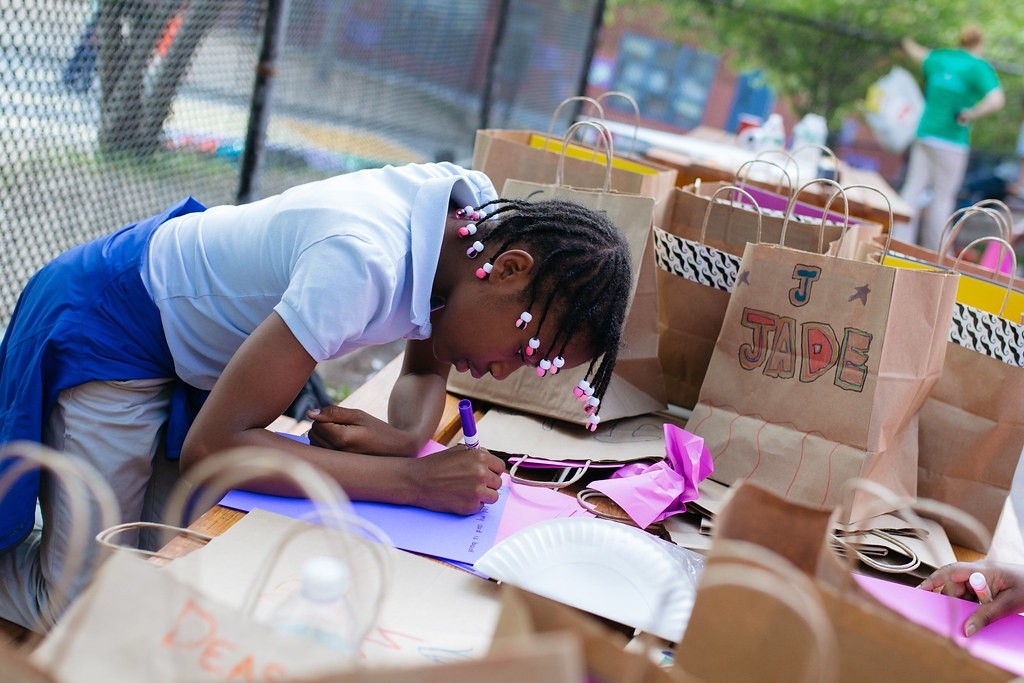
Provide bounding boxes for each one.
[968,569,993,605]
[458,397,480,452]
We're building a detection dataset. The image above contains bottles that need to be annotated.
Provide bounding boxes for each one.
[785,113,828,189]
[752,114,786,182]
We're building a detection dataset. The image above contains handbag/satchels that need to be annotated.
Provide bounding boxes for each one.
[447,93,1024,550]
[2,440,1024,683]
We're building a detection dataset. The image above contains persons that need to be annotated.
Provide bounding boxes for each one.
[891,24,1005,257]
[0,159,632,637]
[917,559,1024,638]
